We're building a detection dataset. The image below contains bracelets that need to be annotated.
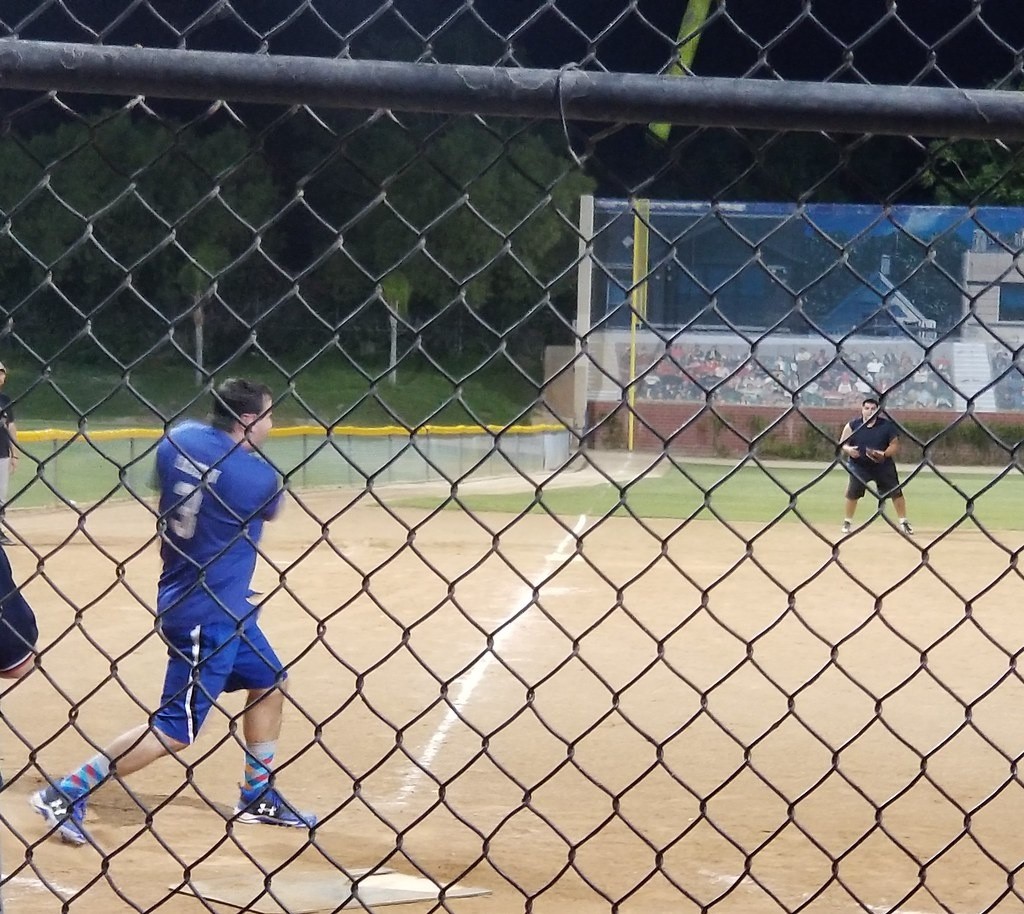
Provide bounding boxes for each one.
[12,456,19,459]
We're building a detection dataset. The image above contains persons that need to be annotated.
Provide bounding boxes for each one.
[0,360,19,546]
[28,376,318,848]
[838,398,914,535]
[0,540,39,680]
[619,341,955,409]
[992,348,1023,412]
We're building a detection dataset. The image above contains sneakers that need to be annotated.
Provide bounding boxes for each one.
[841,520,850,533]
[28,778,88,844]
[900,520,914,536]
[234,781,316,829]
